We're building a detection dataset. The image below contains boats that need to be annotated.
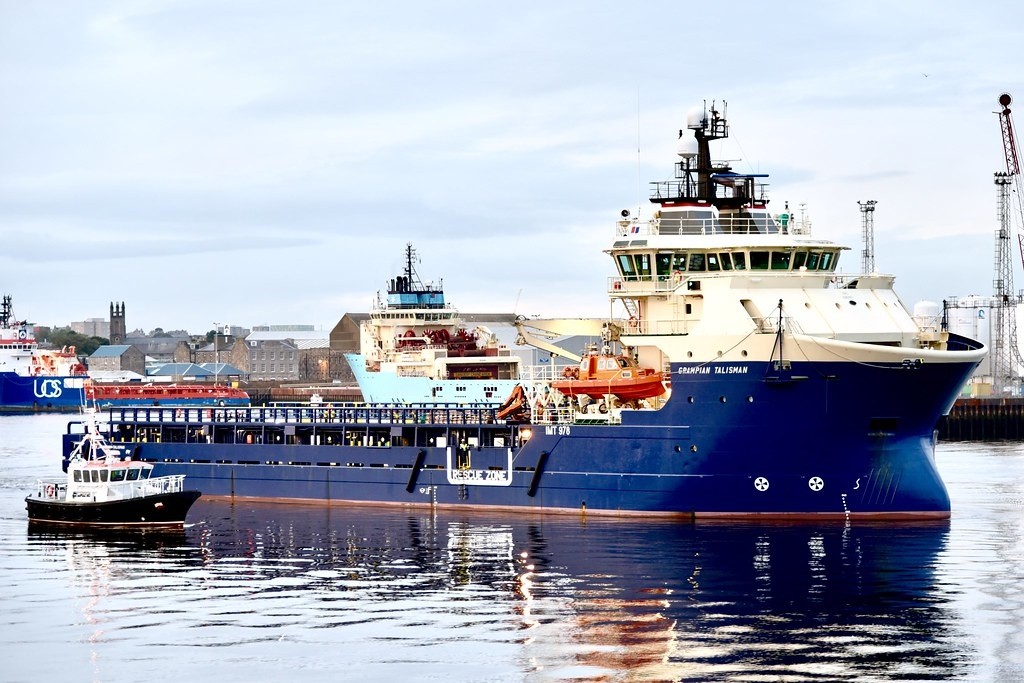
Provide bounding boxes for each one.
[25,384,201,529]
[0,295,252,415]
[62,92,989,526]
[342,240,557,420]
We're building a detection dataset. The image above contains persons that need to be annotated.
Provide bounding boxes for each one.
[460,439,467,466]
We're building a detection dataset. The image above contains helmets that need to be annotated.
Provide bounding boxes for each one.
[461,439,465,441]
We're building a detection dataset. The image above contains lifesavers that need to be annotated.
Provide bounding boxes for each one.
[45,485,54,497]
[629,316,638,326]
[562,367,580,380]
[672,270,683,282]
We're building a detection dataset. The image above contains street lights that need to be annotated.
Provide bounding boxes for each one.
[855,200,878,275]
[214,322,221,384]
